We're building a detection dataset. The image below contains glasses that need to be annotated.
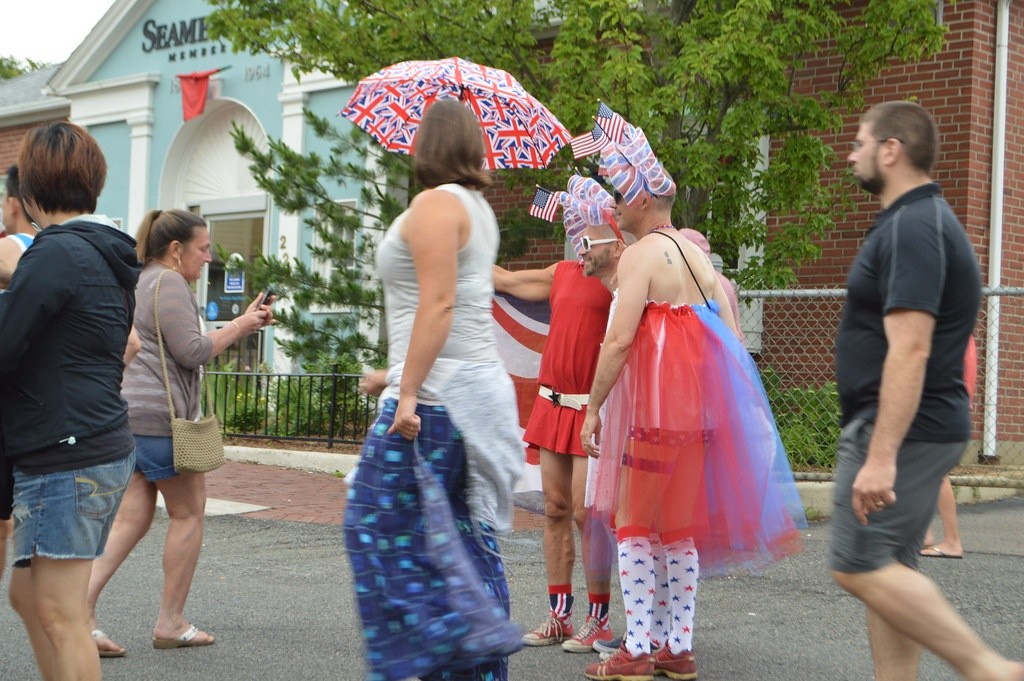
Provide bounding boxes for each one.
[849,136,904,152]
[580,235,627,251]
[613,190,621,204]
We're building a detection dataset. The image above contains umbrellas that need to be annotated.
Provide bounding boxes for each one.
[339,58,573,170]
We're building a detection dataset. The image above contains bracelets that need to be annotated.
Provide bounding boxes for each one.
[233,320,242,341]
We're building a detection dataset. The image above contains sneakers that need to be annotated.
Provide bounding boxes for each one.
[651,640,698,679]
[561,615,615,654]
[521,613,573,647]
[585,642,653,681]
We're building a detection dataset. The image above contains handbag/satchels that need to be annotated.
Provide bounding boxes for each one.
[171,410,228,473]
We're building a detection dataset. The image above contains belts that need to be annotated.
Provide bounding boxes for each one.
[538,385,590,410]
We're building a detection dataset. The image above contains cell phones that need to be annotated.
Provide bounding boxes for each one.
[254,289,275,312]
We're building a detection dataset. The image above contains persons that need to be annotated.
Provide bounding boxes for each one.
[0,120,277,681]
[919,334,977,559]
[357,165,744,681]
[828,100,1024,681]
[376,97,527,681]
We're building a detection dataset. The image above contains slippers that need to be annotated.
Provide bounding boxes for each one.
[152,623,217,649]
[920,546,963,558]
[90,628,129,658]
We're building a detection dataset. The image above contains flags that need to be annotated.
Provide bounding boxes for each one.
[177,64,231,119]
[529,98,627,223]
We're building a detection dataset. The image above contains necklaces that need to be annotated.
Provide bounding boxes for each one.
[650,225,675,233]
